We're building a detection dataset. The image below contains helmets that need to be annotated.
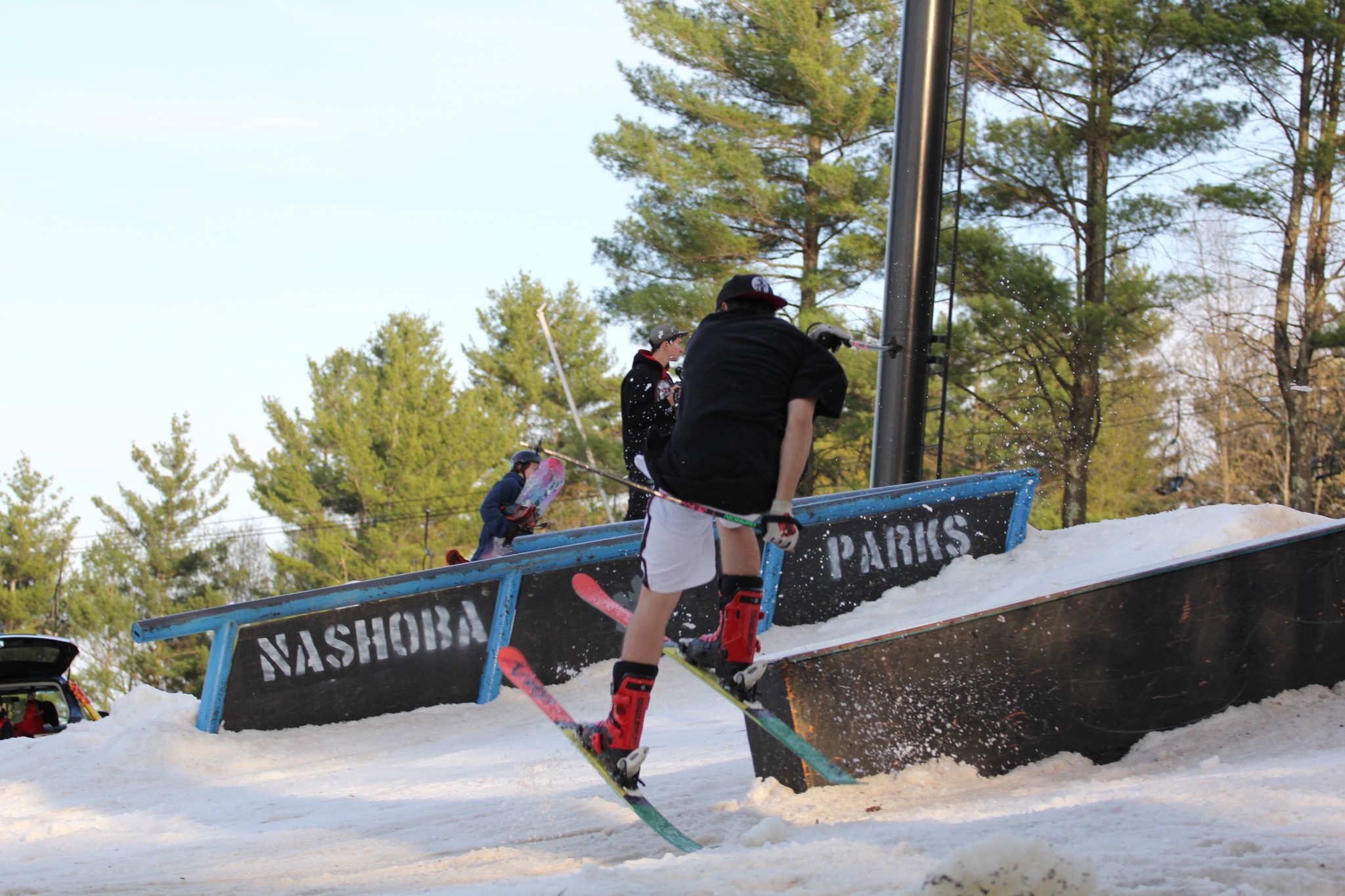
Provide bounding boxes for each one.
[510,450,543,472]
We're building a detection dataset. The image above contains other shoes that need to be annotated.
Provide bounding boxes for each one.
[576,661,659,790]
[684,572,768,703]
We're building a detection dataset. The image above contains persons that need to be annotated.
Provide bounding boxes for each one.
[12,690,45,738]
[576,273,849,790]
[0,696,16,741]
[620,324,688,521]
[471,450,543,561]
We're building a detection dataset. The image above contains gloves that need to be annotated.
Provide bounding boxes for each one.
[507,524,533,538]
[808,323,853,353]
[761,499,799,554]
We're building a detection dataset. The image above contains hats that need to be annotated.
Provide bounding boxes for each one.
[649,324,689,344]
[716,273,787,312]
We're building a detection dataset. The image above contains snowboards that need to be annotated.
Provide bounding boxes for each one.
[471,455,568,564]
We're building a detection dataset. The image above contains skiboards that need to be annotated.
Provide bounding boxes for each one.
[493,573,865,856]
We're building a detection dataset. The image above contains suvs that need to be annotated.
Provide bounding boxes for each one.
[0,632,106,744]
